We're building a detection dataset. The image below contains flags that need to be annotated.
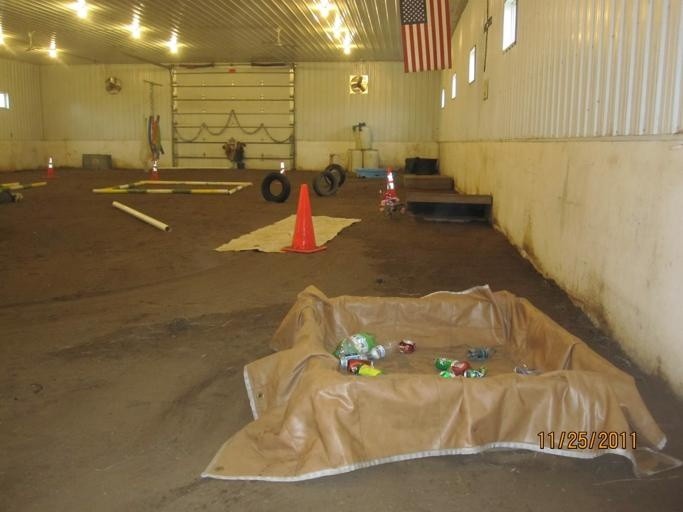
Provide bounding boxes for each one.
[398,0,454,74]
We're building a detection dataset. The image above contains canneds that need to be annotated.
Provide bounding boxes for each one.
[398,338,416,353]
[435,356,490,380]
[340,354,361,369]
[346,359,375,373]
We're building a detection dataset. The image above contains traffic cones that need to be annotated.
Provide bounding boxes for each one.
[149,158,158,181]
[276,161,286,184]
[381,167,399,212]
[283,183,329,253]
[44,156,57,179]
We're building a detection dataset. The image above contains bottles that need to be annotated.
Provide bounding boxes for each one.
[367,341,398,359]
[466,348,496,361]
[339,354,383,376]
[333,331,376,357]
